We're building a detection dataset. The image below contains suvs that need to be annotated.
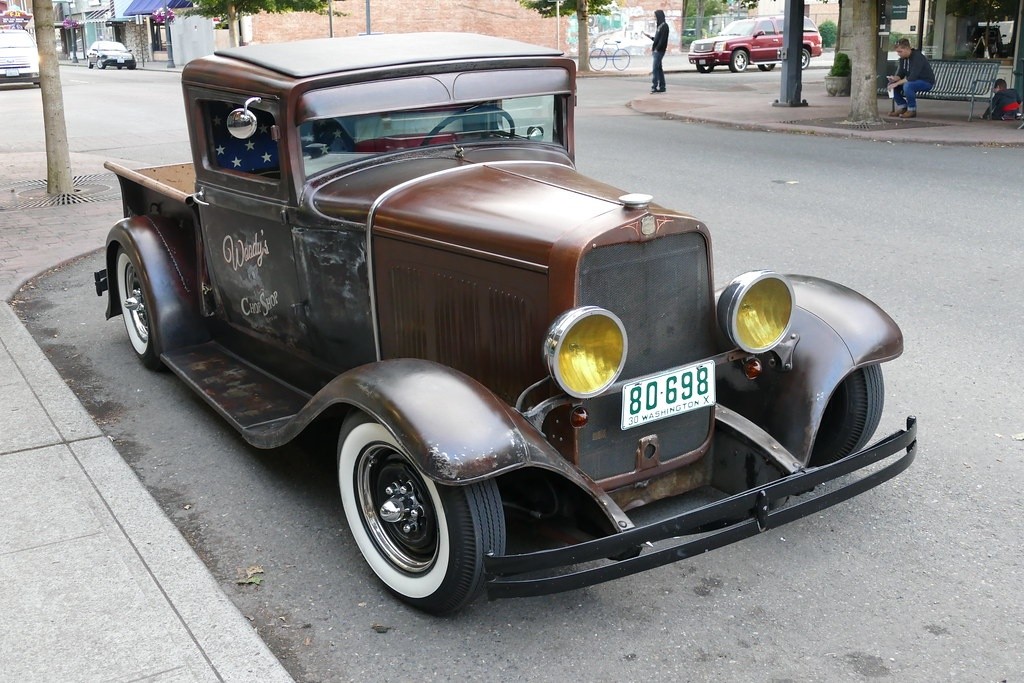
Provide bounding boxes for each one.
[689,17,823,73]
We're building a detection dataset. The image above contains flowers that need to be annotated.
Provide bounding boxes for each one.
[62,16,81,32]
[151,6,175,25]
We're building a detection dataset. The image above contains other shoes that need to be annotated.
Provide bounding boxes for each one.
[899,110,916,119]
[650,87,665,94]
[888,108,907,117]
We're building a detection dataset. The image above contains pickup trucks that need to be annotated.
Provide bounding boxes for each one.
[94,32,918,619]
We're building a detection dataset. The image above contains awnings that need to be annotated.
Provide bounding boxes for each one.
[123,0,193,16]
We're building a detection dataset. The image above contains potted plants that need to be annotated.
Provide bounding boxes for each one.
[823,51,852,97]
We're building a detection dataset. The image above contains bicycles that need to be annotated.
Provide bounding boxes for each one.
[589,39,630,71]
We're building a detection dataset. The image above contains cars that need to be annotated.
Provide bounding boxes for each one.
[971,25,1007,46]
[0,29,41,88]
[87,40,136,70]
[682,29,697,46]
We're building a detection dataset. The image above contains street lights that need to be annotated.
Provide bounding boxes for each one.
[67,0,78,63]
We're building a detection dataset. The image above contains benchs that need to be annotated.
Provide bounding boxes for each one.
[891,58,1002,123]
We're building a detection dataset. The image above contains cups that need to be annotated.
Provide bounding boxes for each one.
[887,89,894,98]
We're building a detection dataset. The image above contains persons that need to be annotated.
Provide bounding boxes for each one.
[642,9,669,93]
[886,38,936,117]
[979,78,1021,121]
